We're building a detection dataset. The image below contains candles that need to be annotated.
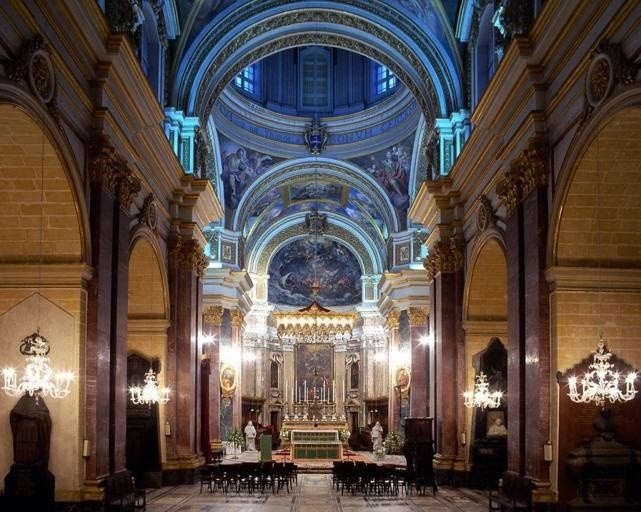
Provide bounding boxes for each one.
[279,377,350,405]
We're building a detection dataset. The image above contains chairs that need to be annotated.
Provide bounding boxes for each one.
[195,446,442,502]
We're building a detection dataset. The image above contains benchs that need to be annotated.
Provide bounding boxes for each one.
[100,465,151,512]
[483,470,536,512]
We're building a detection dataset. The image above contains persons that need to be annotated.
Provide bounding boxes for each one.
[370,421,384,450]
[385,154,408,205]
[224,148,249,202]
[245,421,256,451]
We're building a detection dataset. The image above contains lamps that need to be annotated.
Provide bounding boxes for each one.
[124,231,175,408]
[456,243,506,412]
[2,106,80,405]
[564,126,641,410]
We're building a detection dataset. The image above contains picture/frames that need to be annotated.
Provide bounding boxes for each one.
[291,341,336,406]
